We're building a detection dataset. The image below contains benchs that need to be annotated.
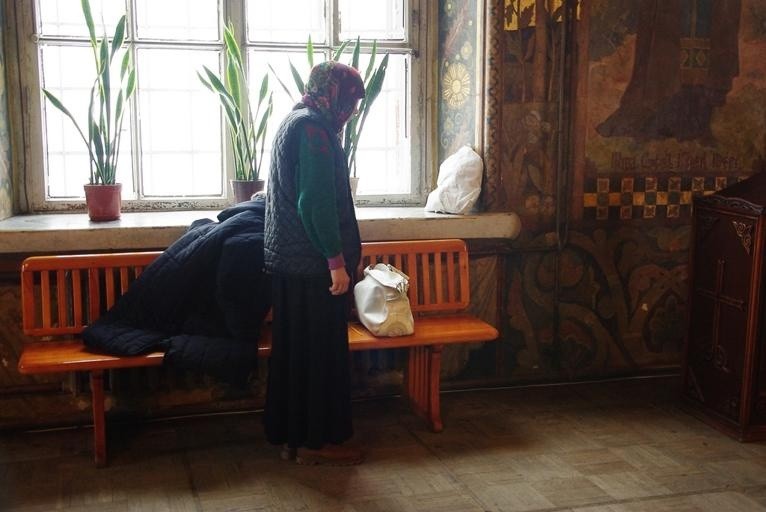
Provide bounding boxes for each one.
[17,238,499,467]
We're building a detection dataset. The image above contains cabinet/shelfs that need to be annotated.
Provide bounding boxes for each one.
[681,175,766,444]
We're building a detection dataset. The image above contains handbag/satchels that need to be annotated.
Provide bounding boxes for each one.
[352,261,420,339]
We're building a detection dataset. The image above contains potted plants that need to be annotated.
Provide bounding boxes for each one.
[41,1,137,221]
[282,35,389,202]
[207,22,273,200]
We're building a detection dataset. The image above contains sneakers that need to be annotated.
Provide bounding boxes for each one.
[279,438,297,462]
[295,437,367,466]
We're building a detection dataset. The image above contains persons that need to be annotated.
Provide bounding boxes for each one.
[261,60,367,467]
